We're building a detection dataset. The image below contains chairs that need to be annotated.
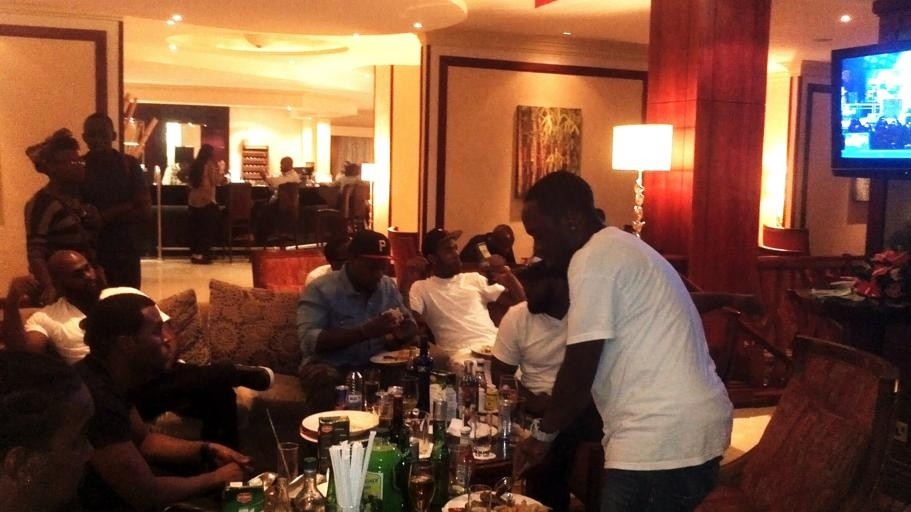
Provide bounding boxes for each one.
[217,179,377,263]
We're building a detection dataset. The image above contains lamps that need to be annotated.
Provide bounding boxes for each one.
[609,123,675,236]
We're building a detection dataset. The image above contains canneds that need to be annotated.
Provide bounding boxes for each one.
[485,388,499,410]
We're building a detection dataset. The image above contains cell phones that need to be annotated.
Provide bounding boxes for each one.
[476,242,491,258]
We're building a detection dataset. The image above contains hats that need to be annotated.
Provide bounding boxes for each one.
[348,231,398,262]
[423,227,462,258]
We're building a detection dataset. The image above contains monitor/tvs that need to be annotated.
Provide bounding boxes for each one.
[830,39,911,181]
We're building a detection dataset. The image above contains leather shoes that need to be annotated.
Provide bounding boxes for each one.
[191,256,210,263]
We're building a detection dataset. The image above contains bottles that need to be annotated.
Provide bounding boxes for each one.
[274,330,526,512]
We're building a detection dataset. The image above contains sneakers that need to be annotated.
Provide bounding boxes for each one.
[236,362,274,391]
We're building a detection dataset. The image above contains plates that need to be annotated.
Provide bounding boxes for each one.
[443,489,545,512]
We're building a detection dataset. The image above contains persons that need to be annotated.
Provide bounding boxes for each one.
[516,170,737,511]
[72,111,152,289]
[234,156,302,241]
[319,159,362,210]
[176,144,226,264]
[3,223,606,511]
[22,127,105,310]
